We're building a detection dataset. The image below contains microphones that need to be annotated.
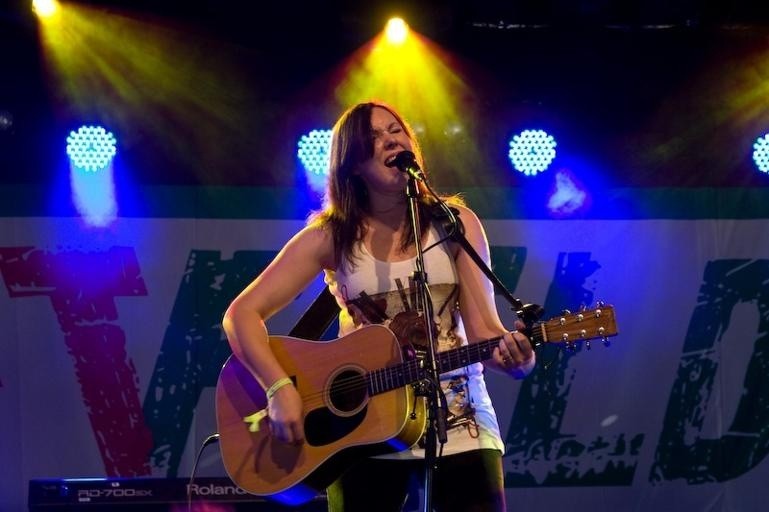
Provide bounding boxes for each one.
[396,150,424,181]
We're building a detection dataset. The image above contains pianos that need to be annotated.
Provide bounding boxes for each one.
[25,475,259,511]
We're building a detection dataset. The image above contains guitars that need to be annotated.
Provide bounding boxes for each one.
[216,302,620,504]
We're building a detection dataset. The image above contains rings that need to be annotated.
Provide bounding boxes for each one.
[502,355,514,364]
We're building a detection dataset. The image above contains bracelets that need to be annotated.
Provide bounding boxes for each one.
[266,376,294,400]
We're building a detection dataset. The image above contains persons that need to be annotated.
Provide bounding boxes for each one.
[221,102,538,512]
